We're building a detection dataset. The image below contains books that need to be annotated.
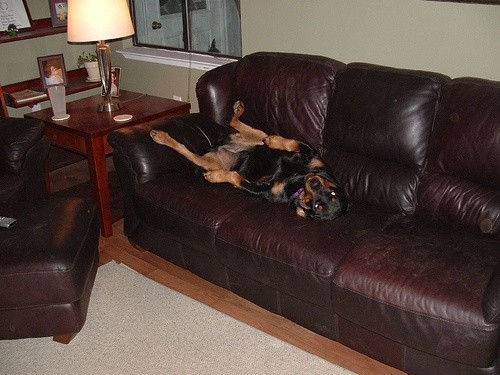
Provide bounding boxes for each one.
[11,89,47,102]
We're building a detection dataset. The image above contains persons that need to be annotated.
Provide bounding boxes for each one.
[58,4,65,21]
[45,64,63,85]
[109,72,118,97]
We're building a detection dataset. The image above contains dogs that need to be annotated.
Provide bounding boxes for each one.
[149,100,353,222]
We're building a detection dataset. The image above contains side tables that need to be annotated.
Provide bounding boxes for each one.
[25,90,191,238]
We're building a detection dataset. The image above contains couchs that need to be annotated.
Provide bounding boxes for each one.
[110,51,499,374]
[1,116,99,342]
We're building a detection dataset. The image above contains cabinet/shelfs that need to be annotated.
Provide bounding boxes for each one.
[1,23,103,109]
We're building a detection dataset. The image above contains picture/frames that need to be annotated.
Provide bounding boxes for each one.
[0,0,34,34]
[101,65,121,98]
[49,0,68,28]
[38,53,69,90]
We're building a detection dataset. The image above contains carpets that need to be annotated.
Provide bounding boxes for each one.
[1,261,358,374]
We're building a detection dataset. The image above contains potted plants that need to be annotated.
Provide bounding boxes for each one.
[6,24,16,36]
[77,52,100,83]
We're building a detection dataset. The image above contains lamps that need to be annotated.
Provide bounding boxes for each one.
[67,0,136,113]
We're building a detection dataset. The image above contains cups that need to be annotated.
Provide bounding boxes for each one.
[48,85,66,119]
